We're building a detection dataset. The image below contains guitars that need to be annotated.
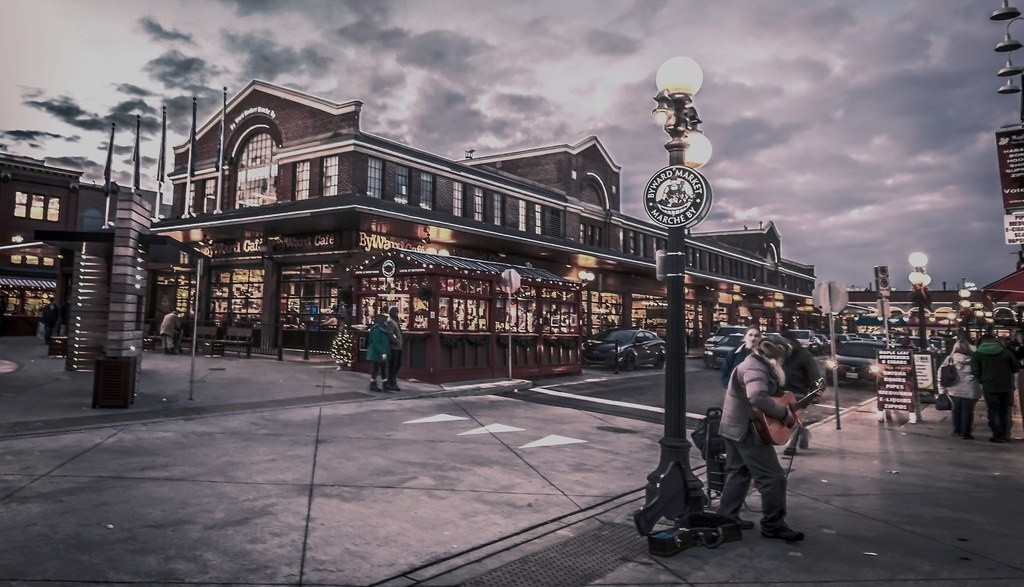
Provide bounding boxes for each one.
[754,377,827,445]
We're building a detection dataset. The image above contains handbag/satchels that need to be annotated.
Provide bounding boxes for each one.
[935,394,952,410]
[37,322,45,340]
[941,358,957,387]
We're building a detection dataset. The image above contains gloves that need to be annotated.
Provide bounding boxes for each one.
[382,353,386,359]
[393,334,396,338]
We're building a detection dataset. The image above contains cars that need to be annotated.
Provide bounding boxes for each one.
[813,332,921,361]
[582,329,665,373]
[703,334,745,366]
[825,340,899,387]
[704,326,748,357]
[788,329,815,350]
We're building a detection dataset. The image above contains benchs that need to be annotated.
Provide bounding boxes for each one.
[144,320,253,358]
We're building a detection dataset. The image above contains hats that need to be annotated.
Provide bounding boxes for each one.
[376,314,387,323]
[744,324,760,336]
[757,332,794,358]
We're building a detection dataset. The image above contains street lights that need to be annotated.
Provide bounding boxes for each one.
[909,252,937,394]
[644,55,713,522]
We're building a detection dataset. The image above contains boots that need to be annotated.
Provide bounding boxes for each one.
[369,378,380,392]
[381,378,395,391]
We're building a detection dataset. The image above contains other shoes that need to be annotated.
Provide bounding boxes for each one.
[1003,435,1010,442]
[989,436,1005,443]
[954,429,963,436]
[784,439,796,455]
[963,434,974,440]
[761,517,805,541]
[736,516,755,530]
[800,428,808,448]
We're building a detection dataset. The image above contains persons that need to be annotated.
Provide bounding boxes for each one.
[387,307,403,391]
[320,310,338,328]
[0,295,6,317]
[365,315,397,392]
[720,325,821,456]
[60,296,71,336]
[1009,338,1024,369]
[719,335,804,542]
[160,310,182,354]
[971,333,1020,444]
[937,339,983,440]
[43,298,59,344]
